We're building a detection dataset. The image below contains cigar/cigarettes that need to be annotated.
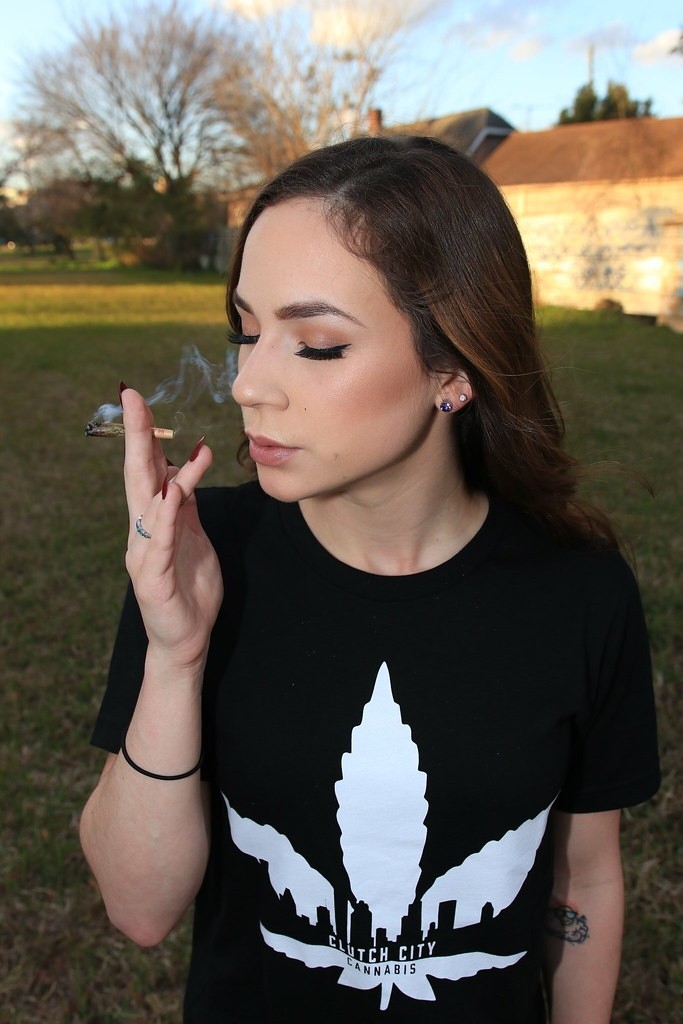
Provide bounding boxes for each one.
[85,422,174,439]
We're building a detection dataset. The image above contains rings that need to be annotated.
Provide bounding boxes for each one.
[135,516,152,539]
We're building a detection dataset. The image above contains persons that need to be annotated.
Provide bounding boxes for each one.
[78,137,662,1024]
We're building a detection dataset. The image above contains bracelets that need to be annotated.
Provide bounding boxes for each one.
[122,737,203,780]
